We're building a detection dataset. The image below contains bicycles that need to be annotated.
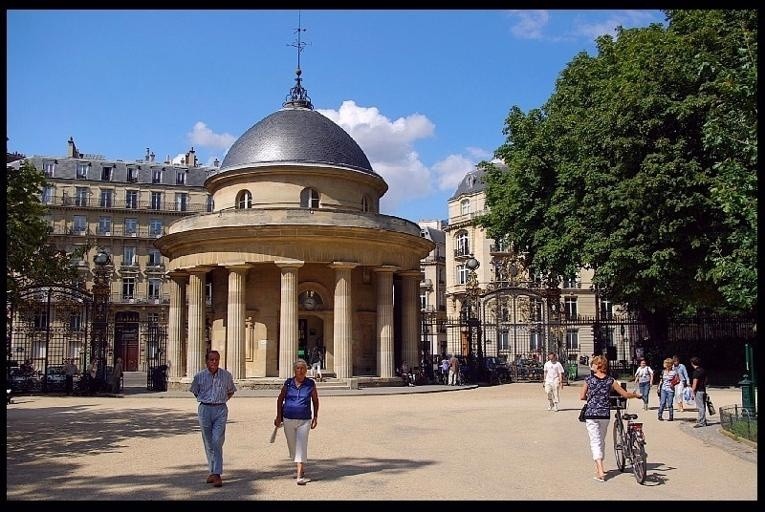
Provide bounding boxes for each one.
[599,391,650,485]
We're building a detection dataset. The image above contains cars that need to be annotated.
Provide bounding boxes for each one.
[434,353,543,384]
[46,366,67,391]
[7,363,26,390]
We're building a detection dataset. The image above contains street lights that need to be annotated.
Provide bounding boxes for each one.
[461,253,480,353]
[89,247,109,378]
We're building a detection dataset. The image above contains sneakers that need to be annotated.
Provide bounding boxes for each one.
[676,404,684,412]
[658,415,664,421]
[547,403,554,411]
[296,475,312,485]
[206,473,223,488]
[554,404,560,411]
[641,404,648,410]
[693,421,708,428]
[667,417,673,421]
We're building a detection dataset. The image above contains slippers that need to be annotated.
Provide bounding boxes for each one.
[593,467,607,482]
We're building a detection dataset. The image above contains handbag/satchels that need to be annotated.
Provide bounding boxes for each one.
[578,404,588,422]
[280,402,285,422]
[671,373,680,385]
[683,387,696,406]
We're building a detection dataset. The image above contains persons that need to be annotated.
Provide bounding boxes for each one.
[671,354,692,412]
[540,350,565,411]
[690,356,710,429]
[188,348,237,489]
[632,359,654,411]
[272,356,320,486]
[447,352,459,386]
[60,358,78,396]
[109,357,126,394]
[580,354,644,482]
[657,356,680,421]
[394,359,416,387]
